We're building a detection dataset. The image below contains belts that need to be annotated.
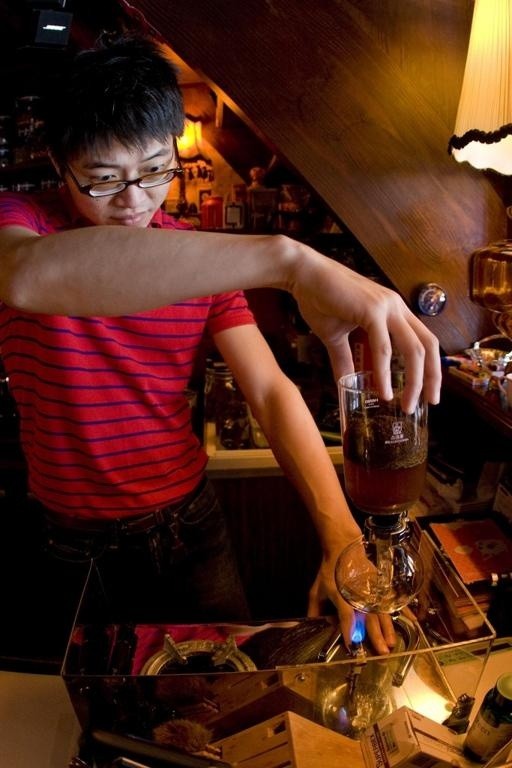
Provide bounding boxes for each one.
[36,489,209,536]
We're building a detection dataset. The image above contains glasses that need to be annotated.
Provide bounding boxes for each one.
[66,137,183,198]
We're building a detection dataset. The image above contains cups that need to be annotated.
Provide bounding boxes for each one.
[496,371,512,407]
[337,371,428,516]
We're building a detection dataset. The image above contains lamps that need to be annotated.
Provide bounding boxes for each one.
[447,0,512,241]
[175,117,215,211]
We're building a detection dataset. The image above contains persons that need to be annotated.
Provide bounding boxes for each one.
[0,38,443,654]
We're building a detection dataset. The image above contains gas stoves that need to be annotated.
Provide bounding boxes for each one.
[140,634,265,725]
